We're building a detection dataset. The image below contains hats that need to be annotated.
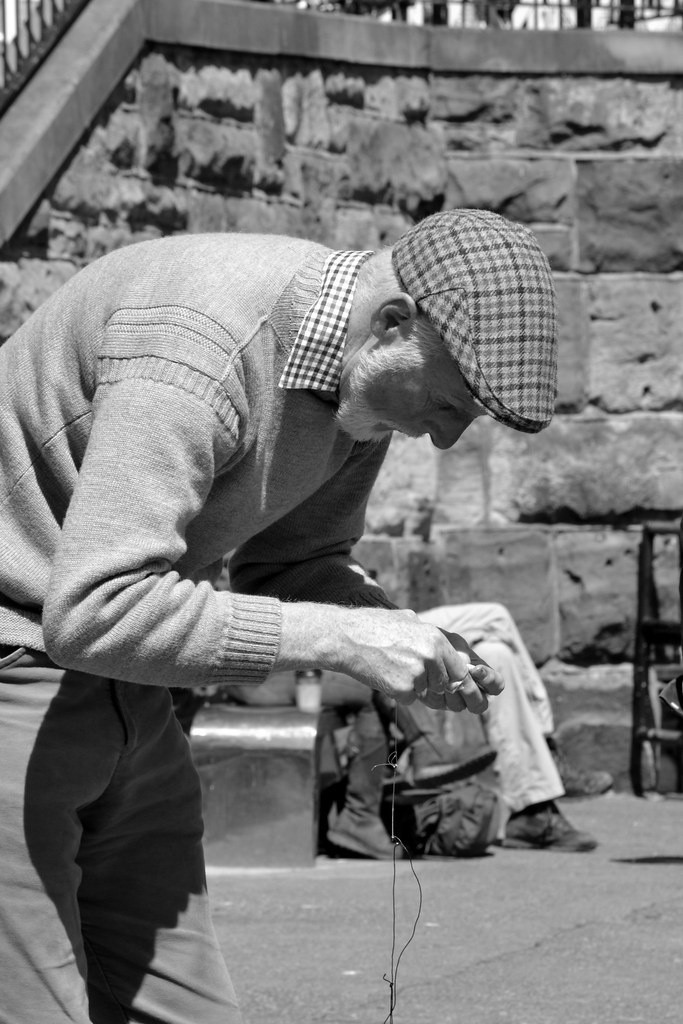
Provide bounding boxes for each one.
[393,209,557,433]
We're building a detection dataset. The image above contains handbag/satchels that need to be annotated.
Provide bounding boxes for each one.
[395,712,503,857]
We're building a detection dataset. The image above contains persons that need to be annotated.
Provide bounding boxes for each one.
[0,207,558,1024]
[212,545,596,860]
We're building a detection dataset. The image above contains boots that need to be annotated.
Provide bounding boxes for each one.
[373,690,497,787]
[327,739,404,861]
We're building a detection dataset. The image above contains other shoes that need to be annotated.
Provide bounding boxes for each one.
[503,800,598,853]
